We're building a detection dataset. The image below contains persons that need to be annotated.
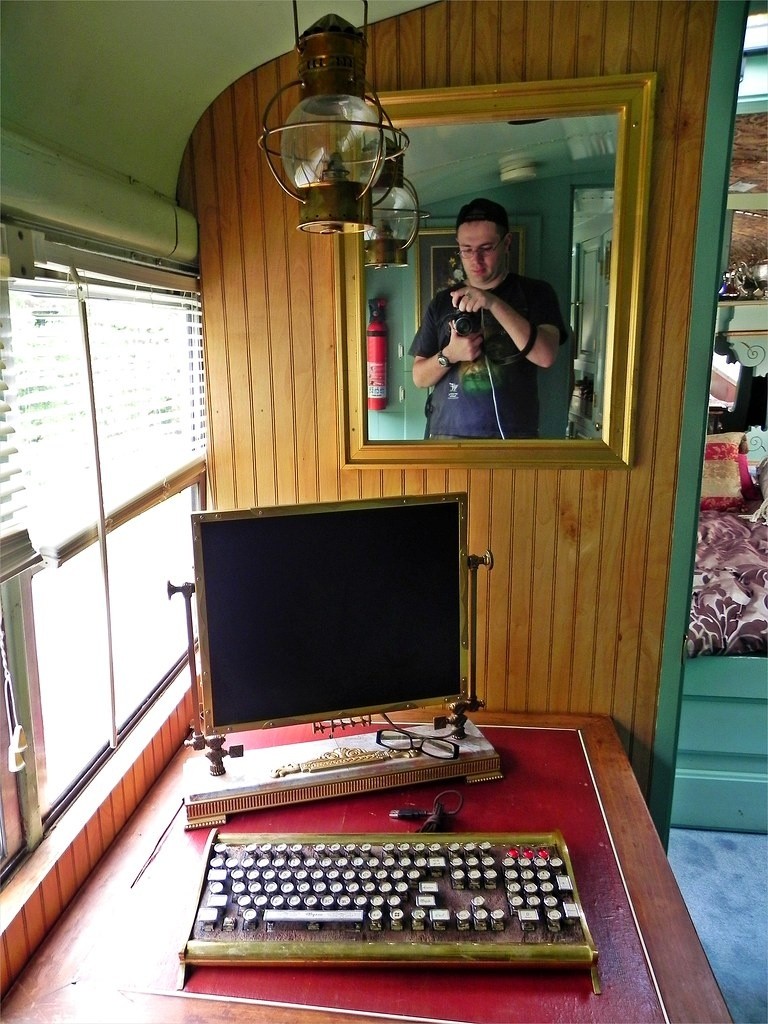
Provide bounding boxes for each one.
[407,198,568,441]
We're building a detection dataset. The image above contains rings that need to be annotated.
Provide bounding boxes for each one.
[466,292,472,299]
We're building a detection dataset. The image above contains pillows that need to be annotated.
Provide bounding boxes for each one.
[698,430,762,515]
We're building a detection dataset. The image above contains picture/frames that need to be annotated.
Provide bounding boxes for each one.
[416,224,526,334]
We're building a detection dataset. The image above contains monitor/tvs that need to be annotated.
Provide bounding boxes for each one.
[192,489,470,737]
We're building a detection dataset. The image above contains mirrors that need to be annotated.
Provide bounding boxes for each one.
[568,184,615,441]
[339,71,658,471]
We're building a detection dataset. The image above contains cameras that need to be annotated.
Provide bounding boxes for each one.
[454,297,477,337]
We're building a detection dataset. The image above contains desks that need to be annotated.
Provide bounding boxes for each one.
[0,713,734,1024]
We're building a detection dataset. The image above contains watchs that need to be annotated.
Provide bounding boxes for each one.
[437,349,457,368]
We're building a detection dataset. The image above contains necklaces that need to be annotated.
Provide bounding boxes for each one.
[491,270,510,291]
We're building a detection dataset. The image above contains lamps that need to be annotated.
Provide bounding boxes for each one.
[363,127,432,271]
[257,0,410,235]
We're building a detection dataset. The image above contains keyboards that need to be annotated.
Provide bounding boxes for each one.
[178,828,601,996]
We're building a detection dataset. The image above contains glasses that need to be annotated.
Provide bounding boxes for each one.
[456,238,502,259]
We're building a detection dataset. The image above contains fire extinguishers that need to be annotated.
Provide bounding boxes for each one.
[366,298,387,409]
